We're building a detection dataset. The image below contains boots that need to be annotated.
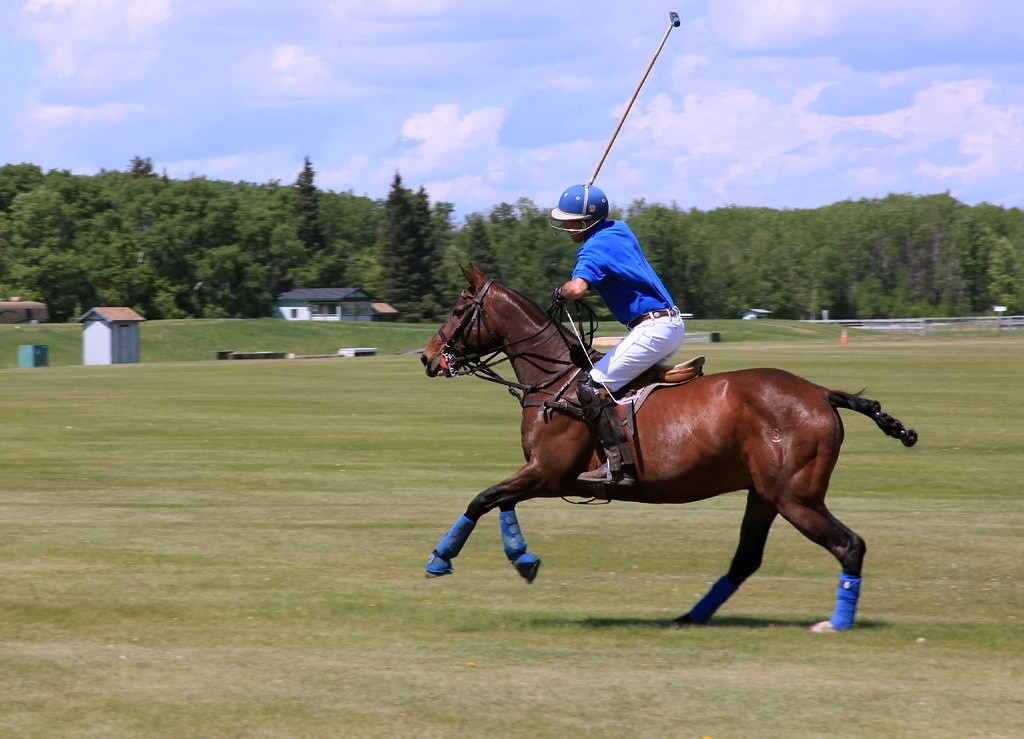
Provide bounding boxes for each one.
[577,388,635,486]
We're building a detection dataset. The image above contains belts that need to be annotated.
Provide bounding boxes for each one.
[626,310,676,330]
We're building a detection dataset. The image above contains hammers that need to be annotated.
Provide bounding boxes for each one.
[587,12,681,186]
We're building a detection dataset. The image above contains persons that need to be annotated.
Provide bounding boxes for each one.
[549,183,686,489]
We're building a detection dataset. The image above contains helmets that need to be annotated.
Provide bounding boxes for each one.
[551,184,609,220]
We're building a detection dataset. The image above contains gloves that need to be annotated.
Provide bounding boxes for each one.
[552,287,568,308]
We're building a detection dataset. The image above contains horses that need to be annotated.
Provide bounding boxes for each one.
[418,261,917,634]
